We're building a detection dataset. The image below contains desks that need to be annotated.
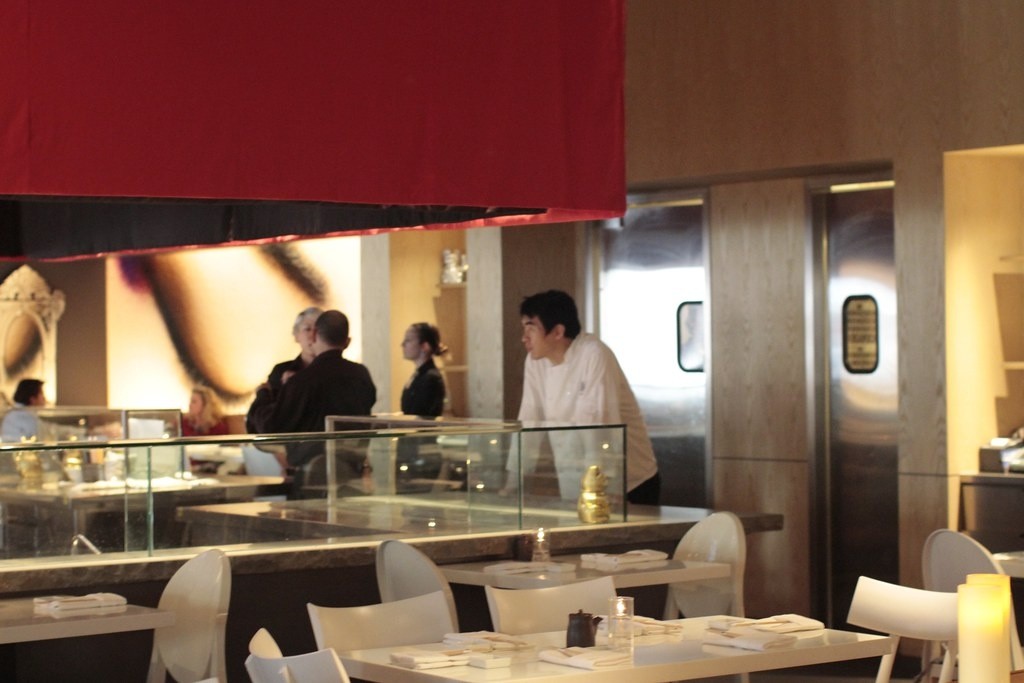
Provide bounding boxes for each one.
[333,614,893,683]
[0,593,177,683]
[437,551,730,591]
[992,551,1023,578]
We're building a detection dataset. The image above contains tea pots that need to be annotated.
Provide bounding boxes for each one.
[565,609,603,648]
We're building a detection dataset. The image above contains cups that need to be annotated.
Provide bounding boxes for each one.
[532,528,550,562]
[609,596,635,638]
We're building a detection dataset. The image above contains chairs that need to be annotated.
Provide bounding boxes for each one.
[147,548,233,683]
[376,540,458,633]
[661,510,749,682]
[486,576,617,636]
[245,627,351,683]
[307,591,456,656]
[846,576,958,683]
[922,529,1024,683]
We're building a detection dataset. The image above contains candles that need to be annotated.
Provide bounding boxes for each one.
[957,583,1011,683]
[966,573,1011,585]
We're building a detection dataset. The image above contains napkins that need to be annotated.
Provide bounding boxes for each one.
[440,631,529,650]
[33,593,128,610]
[747,613,825,634]
[482,560,555,575]
[538,646,632,671]
[388,649,491,670]
[695,624,799,651]
[580,548,669,564]
[592,612,682,637]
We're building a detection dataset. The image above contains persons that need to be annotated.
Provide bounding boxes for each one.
[242,305,323,467]
[394,322,446,491]
[188,387,224,436]
[245,310,377,502]
[0,379,46,442]
[501,290,660,505]
